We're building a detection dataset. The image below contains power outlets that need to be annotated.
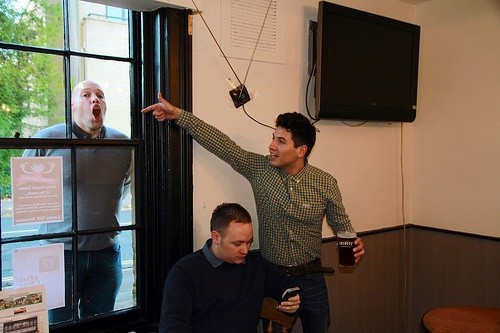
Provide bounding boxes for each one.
[227,84,250,108]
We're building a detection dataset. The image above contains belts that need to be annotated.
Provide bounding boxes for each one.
[273,261,335,279]
[97,246,113,254]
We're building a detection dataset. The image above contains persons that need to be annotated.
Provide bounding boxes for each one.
[159,202,300,333]
[21,80,131,325]
[141,92,365,333]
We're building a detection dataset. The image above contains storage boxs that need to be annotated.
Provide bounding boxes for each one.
[0,287,50,333]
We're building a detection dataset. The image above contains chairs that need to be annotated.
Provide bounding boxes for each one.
[257,298,297,333]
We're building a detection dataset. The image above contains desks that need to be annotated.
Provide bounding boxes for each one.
[420,305,500,333]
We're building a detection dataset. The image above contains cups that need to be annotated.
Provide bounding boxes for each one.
[337,231,356,268]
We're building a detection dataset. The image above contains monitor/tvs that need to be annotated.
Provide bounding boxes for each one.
[315,1,420,123]
